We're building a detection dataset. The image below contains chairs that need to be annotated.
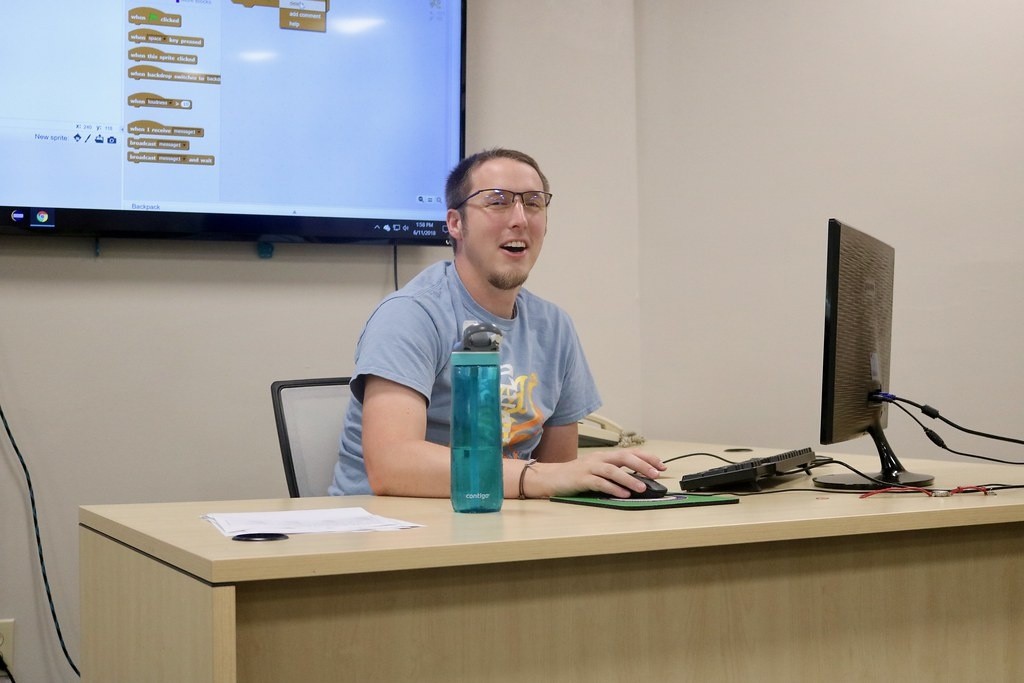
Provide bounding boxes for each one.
[271,376,352,498]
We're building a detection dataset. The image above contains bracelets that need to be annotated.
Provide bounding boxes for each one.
[518,459,541,499]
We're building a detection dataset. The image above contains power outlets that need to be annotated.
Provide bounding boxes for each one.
[0,618,15,677]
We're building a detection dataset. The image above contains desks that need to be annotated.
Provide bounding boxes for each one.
[79,452,1024,683]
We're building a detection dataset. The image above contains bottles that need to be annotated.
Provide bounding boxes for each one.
[450,319,504,512]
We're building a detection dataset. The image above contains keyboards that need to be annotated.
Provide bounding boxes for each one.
[678,447,814,491]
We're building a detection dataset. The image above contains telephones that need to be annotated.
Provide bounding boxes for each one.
[576,412,644,448]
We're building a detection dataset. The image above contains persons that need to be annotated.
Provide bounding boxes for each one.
[328,148,667,500]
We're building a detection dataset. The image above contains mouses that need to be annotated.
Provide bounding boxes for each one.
[580,472,668,500]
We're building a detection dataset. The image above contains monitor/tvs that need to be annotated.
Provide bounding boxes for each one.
[0,0,467,246]
[811,218,935,489]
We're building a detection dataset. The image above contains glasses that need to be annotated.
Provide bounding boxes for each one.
[455,189,553,210]
